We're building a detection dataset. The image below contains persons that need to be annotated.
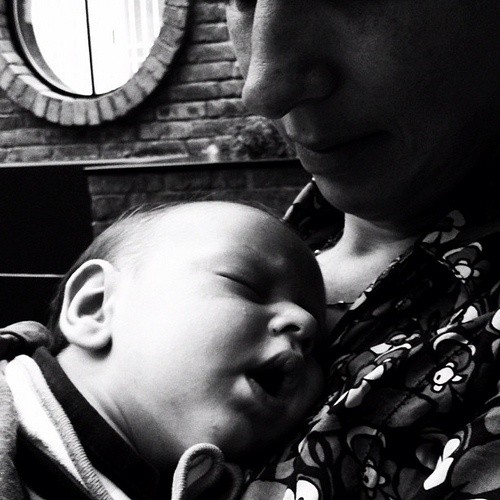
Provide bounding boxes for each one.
[1,0,500,500]
[0,199,328,500]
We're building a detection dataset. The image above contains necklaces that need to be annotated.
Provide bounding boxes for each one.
[311,225,355,307]
